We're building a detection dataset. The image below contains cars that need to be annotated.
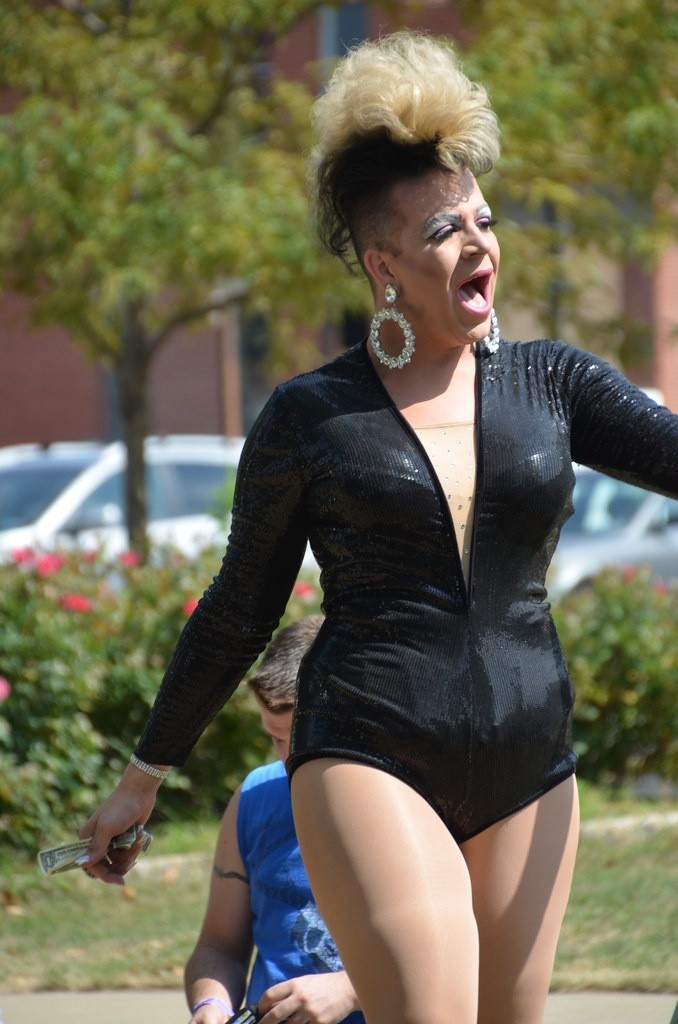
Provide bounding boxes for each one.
[0,439,329,583]
[547,456,677,608]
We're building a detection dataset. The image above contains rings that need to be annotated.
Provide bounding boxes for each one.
[82,853,112,877]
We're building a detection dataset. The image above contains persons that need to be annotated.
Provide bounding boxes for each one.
[72,29,678,1023]
[182,611,365,1024]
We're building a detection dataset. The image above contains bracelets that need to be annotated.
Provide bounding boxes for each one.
[191,997,235,1017]
[127,753,169,780]
